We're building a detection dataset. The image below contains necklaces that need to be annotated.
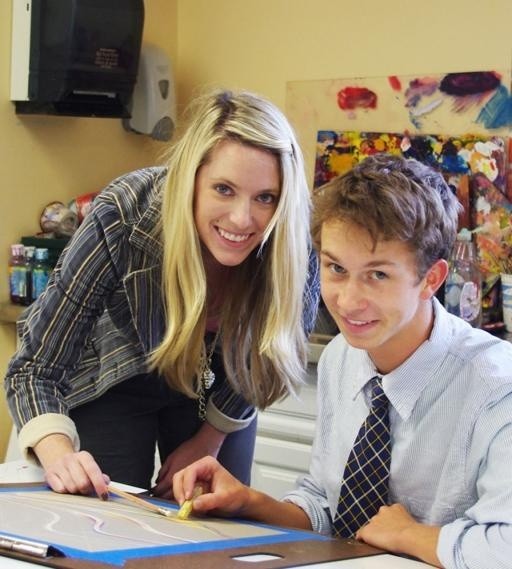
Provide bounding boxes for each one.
[193,267,237,422]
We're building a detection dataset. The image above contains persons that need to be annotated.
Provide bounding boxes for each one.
[3,89,321,501]
[172,155,512,569]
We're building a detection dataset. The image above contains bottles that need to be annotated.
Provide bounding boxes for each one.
[10,244,50,304]
[445,229,483,331]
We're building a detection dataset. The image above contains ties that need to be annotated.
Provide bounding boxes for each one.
[330,376,391,539]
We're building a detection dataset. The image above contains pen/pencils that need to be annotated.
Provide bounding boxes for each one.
[108,485,172,517]
